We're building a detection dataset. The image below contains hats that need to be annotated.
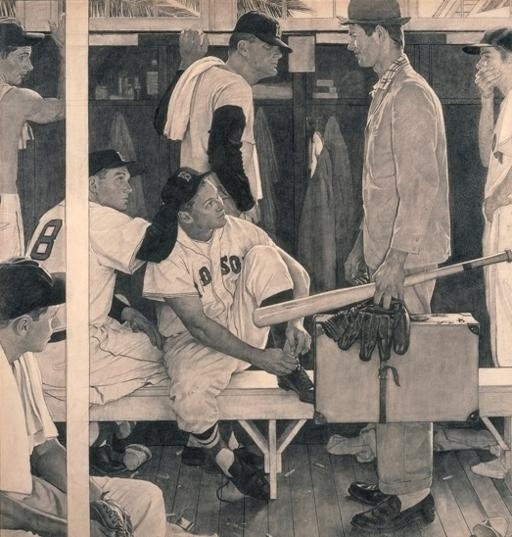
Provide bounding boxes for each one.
[160,167,211,207]
[234,12,292,52]
[0,257,66,320]
[342,0,412,27]
[89,149,135,176]
[462,28,511,55]
[0,21,47,48]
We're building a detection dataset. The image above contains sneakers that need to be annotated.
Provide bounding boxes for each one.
[350,493,435,533]
[470,458,507,481]
[347,482,386,505]
[278,368,314,404]
[181,443,270,501]
[90,444,127,476]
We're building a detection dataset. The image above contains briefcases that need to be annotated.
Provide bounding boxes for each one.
[314,310,481,425]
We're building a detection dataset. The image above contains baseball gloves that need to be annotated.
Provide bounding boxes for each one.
[323,297,410,360]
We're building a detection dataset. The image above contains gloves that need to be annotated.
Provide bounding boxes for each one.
[323,296,410,360]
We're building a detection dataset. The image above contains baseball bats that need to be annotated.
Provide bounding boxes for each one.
[252,249,511,327]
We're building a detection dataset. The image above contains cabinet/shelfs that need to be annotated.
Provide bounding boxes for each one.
[17,29,503,444]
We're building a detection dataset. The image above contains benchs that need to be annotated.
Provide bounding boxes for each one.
[46,364,512,498]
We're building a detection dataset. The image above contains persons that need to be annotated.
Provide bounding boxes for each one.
[140,164,315,503]
[458,27,511,480]
[1,258,168,536]
[24,145,193,476]
[150,9,296,468]
[1,16,68,263]
[338,1,454,536]
